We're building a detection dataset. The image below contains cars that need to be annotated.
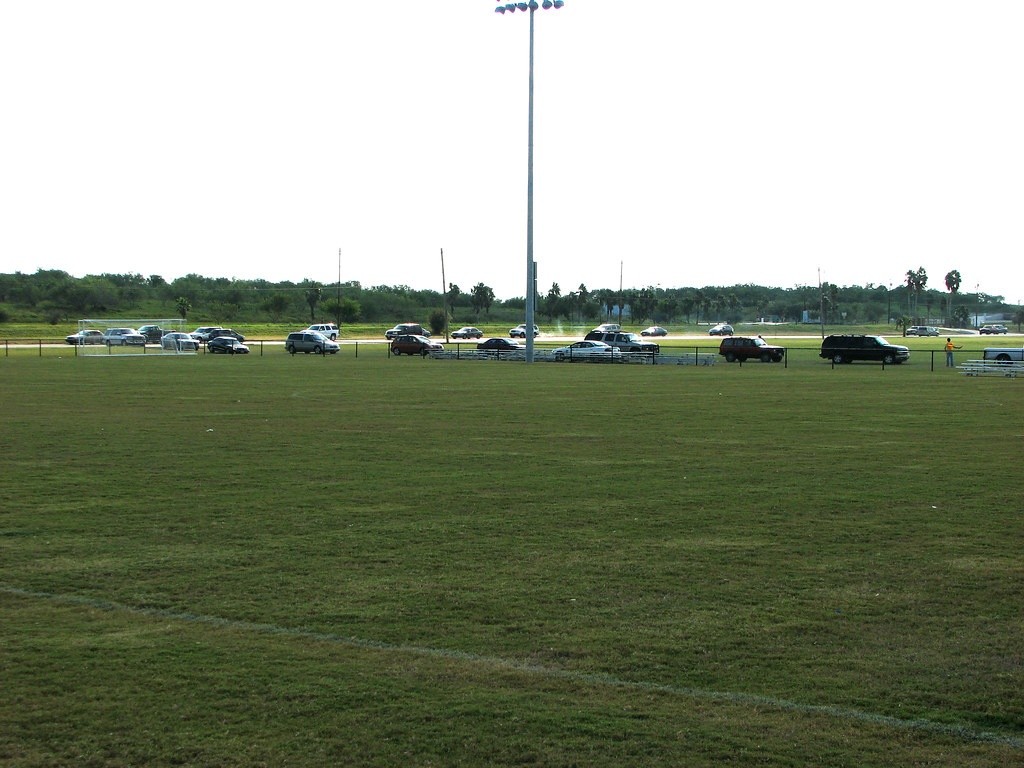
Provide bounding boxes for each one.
[65,324,251,354]
[477,337,527,356]
[980,324,1008,335]
[708,324,735,336]
[421,327,432,338]
[551,339,622,364]
[640,327,668,337]
[451,327,484,339]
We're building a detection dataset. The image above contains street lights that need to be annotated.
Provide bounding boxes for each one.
[494,0,565,361]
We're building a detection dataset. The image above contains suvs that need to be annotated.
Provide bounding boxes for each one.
[906,325,920,335]
[390,333,445,357]
[593,323,622,335]
[918,325,940,337]
[385,324,422,340]
[585,328,660,357]
[509,323,540,339]
[285,330,341,354]
[301,323,340,341]
[719,336,785,363]
[819,333,910,365]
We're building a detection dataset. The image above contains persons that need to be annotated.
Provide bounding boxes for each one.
[944,338,955,367]
[866,339,874,346]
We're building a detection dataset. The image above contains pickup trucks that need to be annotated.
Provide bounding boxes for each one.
[984,345,1024,367]
